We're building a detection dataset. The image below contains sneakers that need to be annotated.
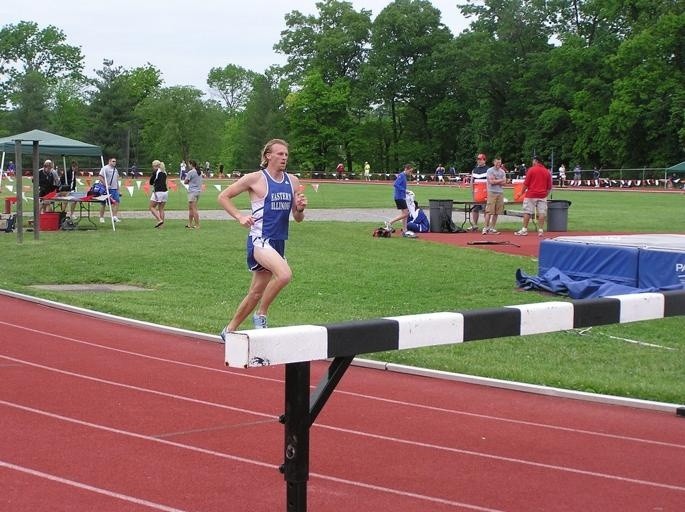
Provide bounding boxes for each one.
[114,216,121,222]
[186,225,200,228]
[220,326,228,341]
[385,220,392,227]
[155,220,164,228]
[514,229,528,236]
[468,224,497,235]
[100,218,105,223]
[253,311,268,328]
[538,232,543,236]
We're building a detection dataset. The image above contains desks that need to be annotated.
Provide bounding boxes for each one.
[454,200,540,233]
[39,196,108,231]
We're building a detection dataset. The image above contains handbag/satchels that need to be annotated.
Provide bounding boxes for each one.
[373,227,391,238]
[87,183,106,197]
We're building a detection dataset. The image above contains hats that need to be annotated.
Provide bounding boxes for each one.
[531,155,544,162]
[477,154,485,160]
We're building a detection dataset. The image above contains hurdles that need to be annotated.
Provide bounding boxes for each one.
[224,289,684,511]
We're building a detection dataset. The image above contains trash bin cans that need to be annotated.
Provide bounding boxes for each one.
[546,200,571,232]
[428,199,453,233]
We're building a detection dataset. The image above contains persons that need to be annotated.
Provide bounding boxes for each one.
[98,157,121,223]
[336,153,600,237]
[179,159,186,180]
[0,156,78,224]
[131,161,138,179]
[218,162,225,177]
[218,138,308,342]
[205,159,210,175]
[184,159,202,229]
[148,159,168,228]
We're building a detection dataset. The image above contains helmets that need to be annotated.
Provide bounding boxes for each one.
[43,160,53,168]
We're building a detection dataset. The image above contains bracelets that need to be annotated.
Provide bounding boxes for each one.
[295,205,305,214]
